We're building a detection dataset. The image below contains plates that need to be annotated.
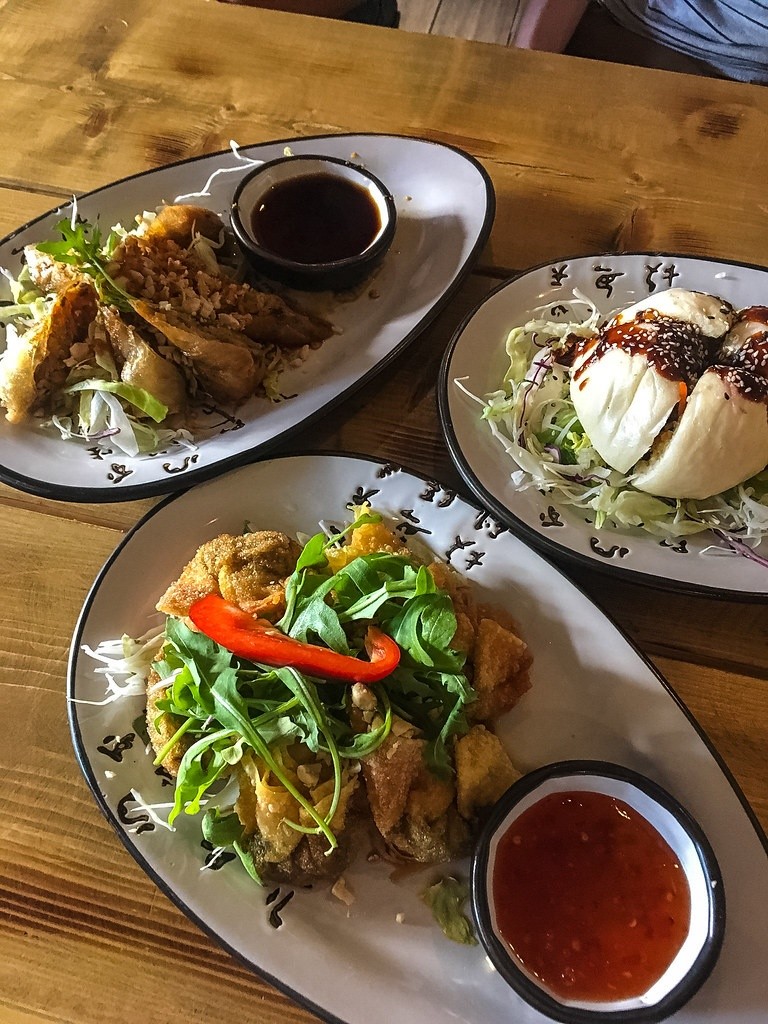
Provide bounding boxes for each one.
[65,452,768,1023]
[1,133,496,506]
[438,250,768,602]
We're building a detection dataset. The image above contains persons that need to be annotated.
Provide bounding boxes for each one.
[516,0,768,87]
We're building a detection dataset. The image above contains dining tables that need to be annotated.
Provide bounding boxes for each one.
[0,1,768,1023]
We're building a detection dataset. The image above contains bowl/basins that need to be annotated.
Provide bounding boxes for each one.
[232,155,397,290]
[472,759,727,1023]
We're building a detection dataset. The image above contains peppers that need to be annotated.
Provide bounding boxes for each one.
[189,598,399,682]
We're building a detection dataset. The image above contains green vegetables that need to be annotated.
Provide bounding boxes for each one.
[143,516,477,886]
[29,215,135,310]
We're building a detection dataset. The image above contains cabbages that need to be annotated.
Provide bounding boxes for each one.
[457,290,768,557]
